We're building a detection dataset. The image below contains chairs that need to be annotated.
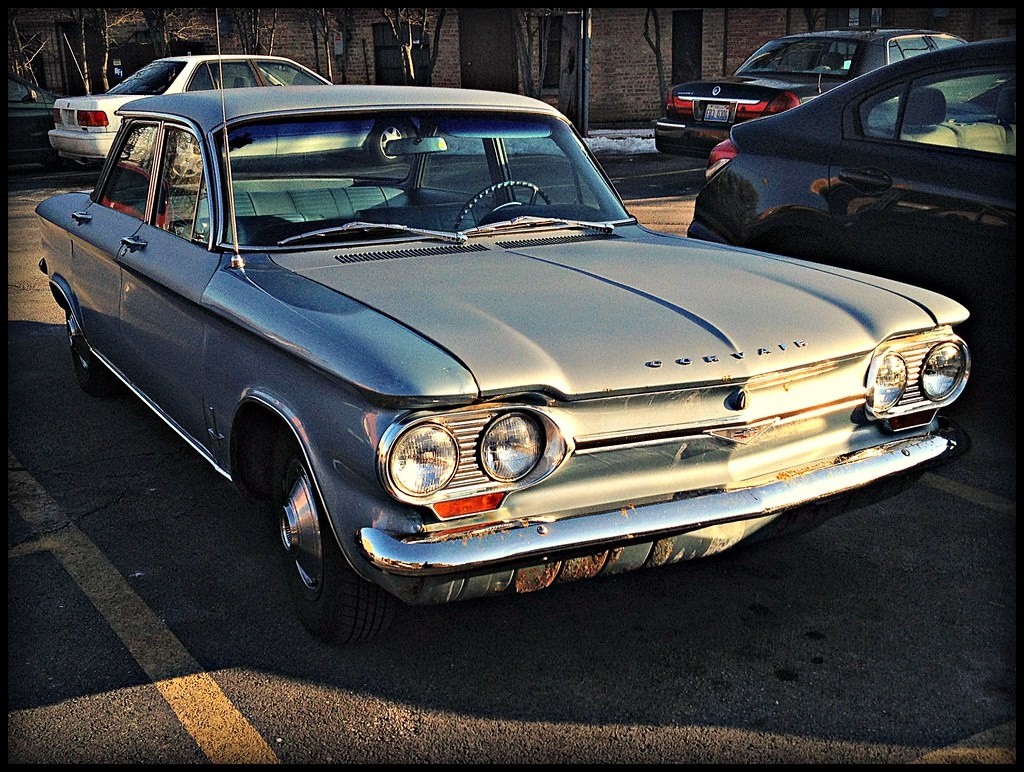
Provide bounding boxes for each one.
[822,52,844,69]
[233,76,247,87]
[900,84,1017,155]
[354,203,476,233]
[204,214,326,246]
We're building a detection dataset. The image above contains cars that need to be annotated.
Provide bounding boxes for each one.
[685,35,1024,420]
[48,52,415,184]
[653,26,989,158]
[7,72,81,170]
[28,82,973,649]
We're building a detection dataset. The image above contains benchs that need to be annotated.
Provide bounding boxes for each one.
[194,186,410,239]
